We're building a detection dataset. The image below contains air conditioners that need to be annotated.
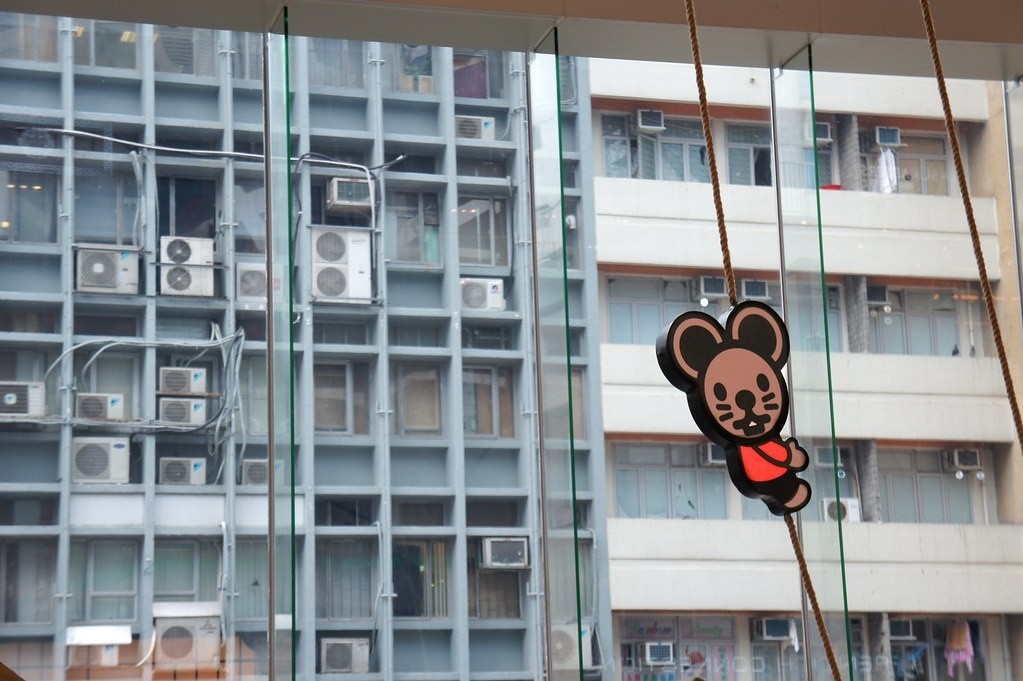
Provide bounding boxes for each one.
[806,446,842,471]
[865,285,891,310]
[310,228,373,306]
[820,498,860,523]
[456,116,496,140]
[751,617,790,643]
[75,248,139,295]
[634,643,673,667]
[804,121,831,147]
[697,443,726,469]
[325,180,374,215]
[689,276,728,304]
[941,449,981,474]
[0,368,529,674]
[632,109,665,133]
[458,278,503,312]
[865,126,900,154]
[735,279,770,303]
[236,263,268,301]
[159,235,213,298]
